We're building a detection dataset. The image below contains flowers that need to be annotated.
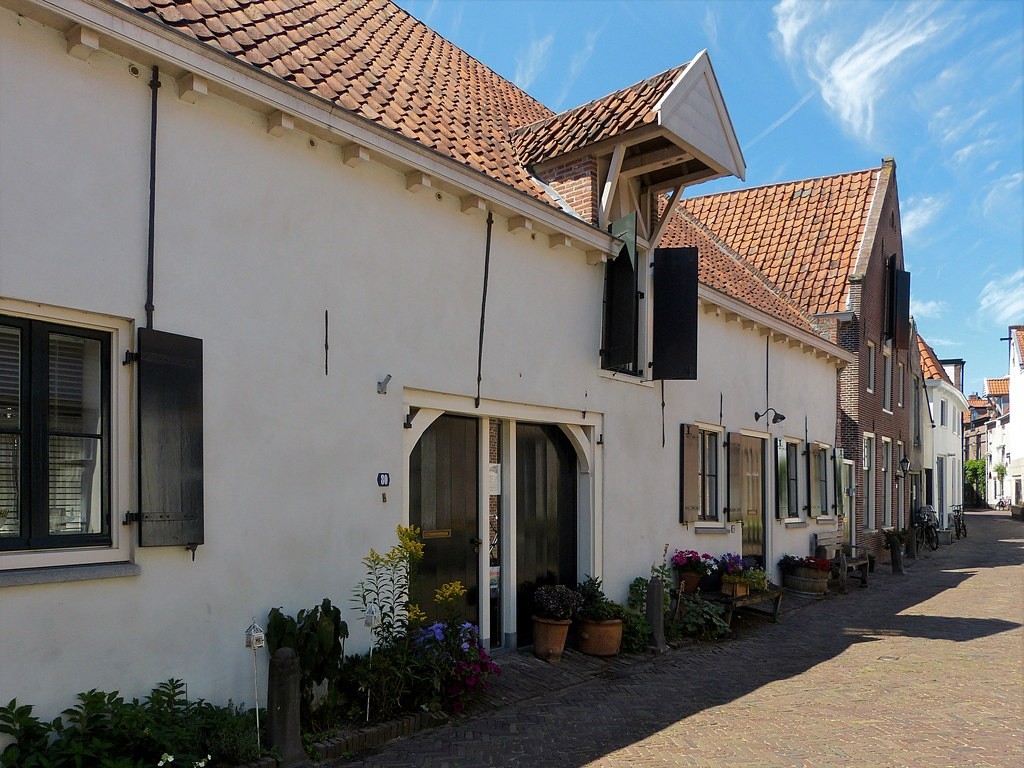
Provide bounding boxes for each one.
[670,548,719,576]
[713,552,770,591]
[533,585,585,620]
[778,552,834,573]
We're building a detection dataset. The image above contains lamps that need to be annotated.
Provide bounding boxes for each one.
[755,408,786,424]
[895,452,911,482]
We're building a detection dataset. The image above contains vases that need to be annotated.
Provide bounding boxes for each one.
[721,583,750,597]
[678,572,702,595]
[532,616,572,664]
[783,566,830,593]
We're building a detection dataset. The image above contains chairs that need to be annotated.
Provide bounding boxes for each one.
[815,531,871,594]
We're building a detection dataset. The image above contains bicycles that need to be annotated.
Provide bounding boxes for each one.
[950,503,967,540]
[916,510,939,554]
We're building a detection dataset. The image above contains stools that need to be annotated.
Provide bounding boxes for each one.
[938,530,953,545]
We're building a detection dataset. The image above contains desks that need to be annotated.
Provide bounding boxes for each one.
[1009,506,1024,520]
[669,583,785,633]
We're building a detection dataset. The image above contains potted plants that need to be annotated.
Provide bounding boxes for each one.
[575,574,628,658]
[859,553,876,572]
